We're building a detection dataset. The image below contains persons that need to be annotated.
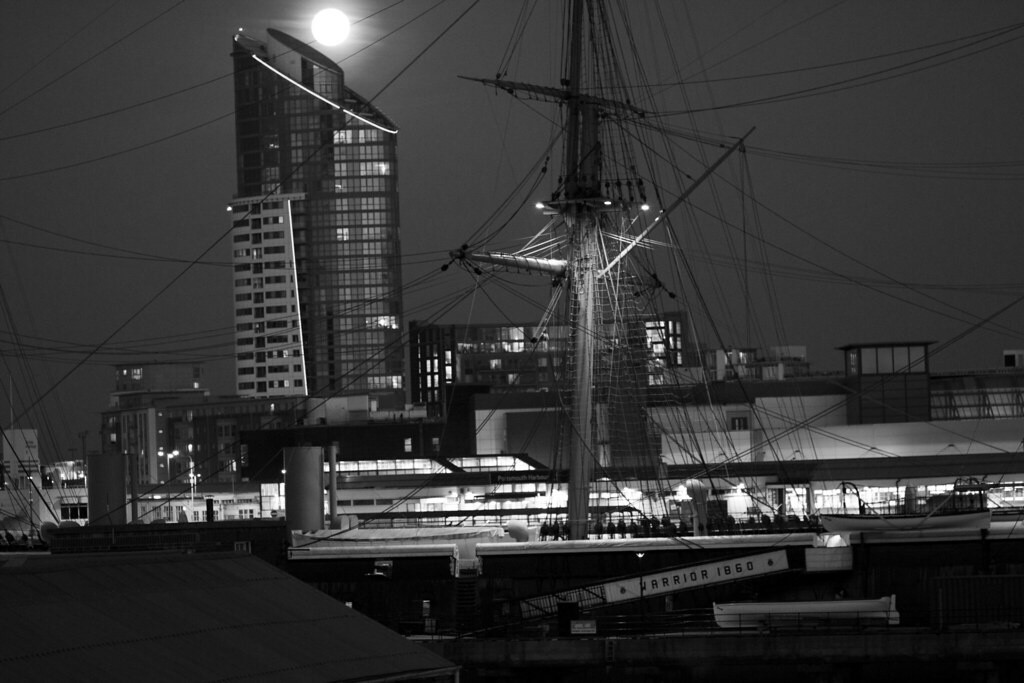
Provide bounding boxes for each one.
[540,520,566,540]
[593,514,689,538]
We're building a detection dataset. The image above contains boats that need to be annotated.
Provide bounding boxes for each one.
[290,0,1024,683]
